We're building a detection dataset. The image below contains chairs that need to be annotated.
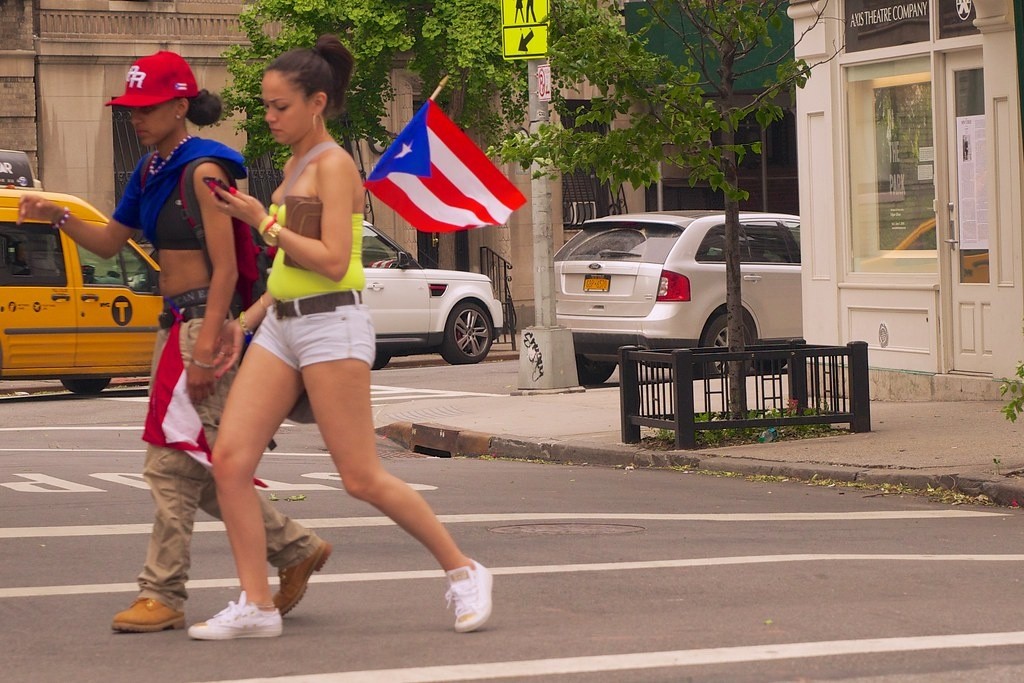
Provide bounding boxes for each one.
[55,248,66,274]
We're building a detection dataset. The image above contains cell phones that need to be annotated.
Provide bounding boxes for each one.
[203,177,231,204]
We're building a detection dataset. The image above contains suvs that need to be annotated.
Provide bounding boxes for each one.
[554,209,801,387]
[130,219,504,369]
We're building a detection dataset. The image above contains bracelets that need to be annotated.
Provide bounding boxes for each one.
[50,206,69,229]
[260,294,267,315]
[258,216,278,236]
[240,311,254,336]
[189,357,218,369]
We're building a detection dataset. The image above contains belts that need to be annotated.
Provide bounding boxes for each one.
[270,290,363,320]
[159,306,229,329]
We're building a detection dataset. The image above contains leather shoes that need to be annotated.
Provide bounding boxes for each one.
[111,597,185,632]
[271,540,333,616]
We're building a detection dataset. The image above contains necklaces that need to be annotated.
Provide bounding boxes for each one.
[149,135,192,176]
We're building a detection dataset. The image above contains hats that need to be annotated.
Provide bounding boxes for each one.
[104,50,198,107]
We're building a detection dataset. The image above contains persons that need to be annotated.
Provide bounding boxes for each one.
[16,49,333,632]
[187,34,493,640]
[10,240,32,275]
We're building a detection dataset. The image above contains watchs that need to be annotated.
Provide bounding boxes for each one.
[263,221,282,246]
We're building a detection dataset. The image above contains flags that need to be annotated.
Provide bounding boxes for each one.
[363,98,528,231]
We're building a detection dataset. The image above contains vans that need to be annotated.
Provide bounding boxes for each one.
[0,148,163,396]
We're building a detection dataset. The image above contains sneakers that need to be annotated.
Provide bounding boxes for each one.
[445,558,493,633]
[188,590,283,640]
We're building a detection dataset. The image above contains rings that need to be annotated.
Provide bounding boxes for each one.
[219,352,226,356]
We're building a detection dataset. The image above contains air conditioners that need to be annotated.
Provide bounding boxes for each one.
[563,201,596,225]
